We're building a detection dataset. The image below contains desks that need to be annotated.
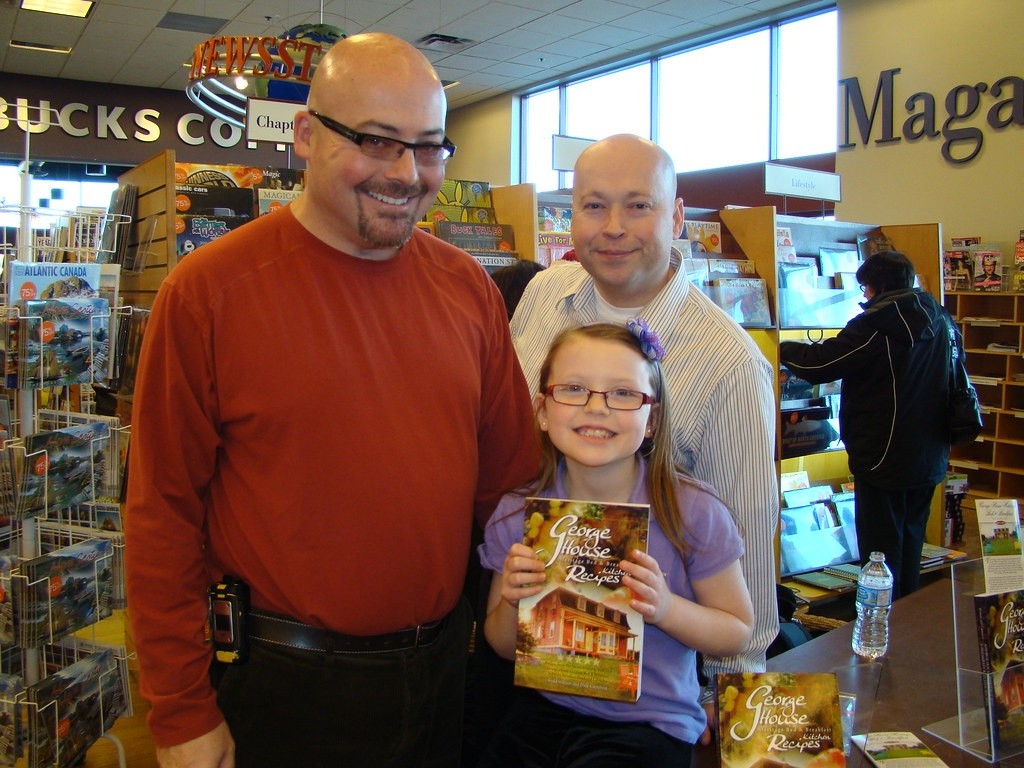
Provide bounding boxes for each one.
[765,577,1024,768]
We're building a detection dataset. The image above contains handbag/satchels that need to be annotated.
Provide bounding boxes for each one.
[940,307,983,446]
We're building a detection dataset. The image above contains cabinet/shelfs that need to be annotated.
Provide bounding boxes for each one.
[943,289,1024,531]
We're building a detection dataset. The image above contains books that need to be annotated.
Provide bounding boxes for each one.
[169,161,517,279]
[713,672,845,768]
[0,182,155,768]
[533,203,772,331]
[513,495,652,703]
[975,587,1024,754]
[775,227,967,593]
[939,233,1024,411]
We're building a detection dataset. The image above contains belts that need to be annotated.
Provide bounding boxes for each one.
[243,612,448,656]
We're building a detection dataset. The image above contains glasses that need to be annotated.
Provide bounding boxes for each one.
[308,107,456,167]
[860,283,872,292]
[543,382,656,411]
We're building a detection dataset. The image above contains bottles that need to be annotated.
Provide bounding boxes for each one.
[851,551,893,659]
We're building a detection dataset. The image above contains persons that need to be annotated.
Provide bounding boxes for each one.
[975,254,1001,291]
[477,322,753,768]
[952,259,971,291]
[488,260,546,323]
[463,134,781,768]
[781,249,952,605]
[121,34,545,767]
